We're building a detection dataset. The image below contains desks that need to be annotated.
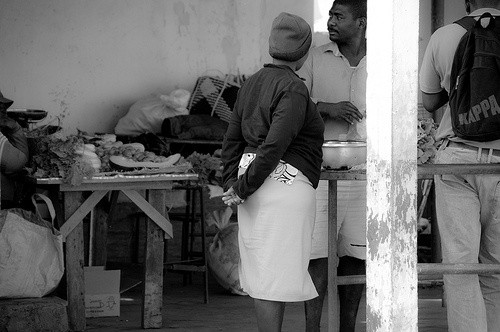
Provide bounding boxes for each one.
[36,173,200,332]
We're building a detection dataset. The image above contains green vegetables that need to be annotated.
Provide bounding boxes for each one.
[417,117,445,165]
[185,151,214,185]
[23,121,116,178]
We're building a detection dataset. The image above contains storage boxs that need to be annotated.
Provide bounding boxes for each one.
[83,266,121,318]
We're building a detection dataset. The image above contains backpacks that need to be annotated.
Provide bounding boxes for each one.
[449,12,500,142]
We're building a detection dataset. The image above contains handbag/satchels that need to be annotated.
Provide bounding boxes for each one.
[0,193,65,298]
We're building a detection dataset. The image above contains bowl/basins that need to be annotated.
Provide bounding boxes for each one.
[321,141,366,173]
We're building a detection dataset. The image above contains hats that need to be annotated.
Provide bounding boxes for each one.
[269,12,312,61]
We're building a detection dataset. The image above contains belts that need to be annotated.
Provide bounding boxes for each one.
[441,140,500,157]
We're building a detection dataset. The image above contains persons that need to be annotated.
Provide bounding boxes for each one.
[0,91,28,172]
[222,11,326,332]
[419,0,500,332]
[299,0,369,331]
